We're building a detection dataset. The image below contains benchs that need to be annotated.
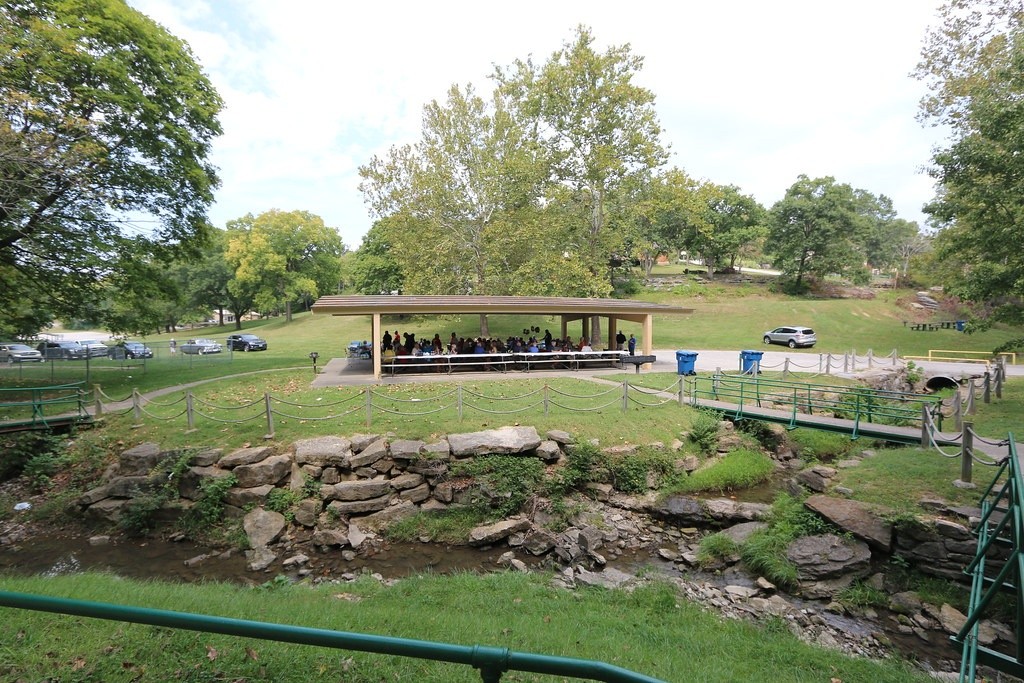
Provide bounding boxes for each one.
[935,324,942,327]
[910,326,922,330]
[929,326,941,331]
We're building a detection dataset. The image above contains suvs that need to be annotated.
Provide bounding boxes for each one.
[763,326,816,348]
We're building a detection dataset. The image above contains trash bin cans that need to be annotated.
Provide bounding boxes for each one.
[740,350,763,374]
[676,350,698,375]
[957,320,965,331]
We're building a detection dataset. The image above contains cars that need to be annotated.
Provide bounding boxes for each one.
[180,338,223,356]
[226,333,267,352]
[0,342,41,364]
[72,339,107,357]
[37,338,84,360]
[107,341,153,360]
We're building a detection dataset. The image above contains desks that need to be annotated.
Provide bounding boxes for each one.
[916,324,931,331]
[941,321,956,329]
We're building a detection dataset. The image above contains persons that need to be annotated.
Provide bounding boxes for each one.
[394,331,400,343]
[576,337,598,359]
[169,339,176,354]
[628,334,636,355]
[506,336,526,352]
[527,335,539,352]
[360,341,372,359]
[446,332,506,367]
[384,332,442,372]
[540,330,552,360]
[552,336,573,359]
[383,331,392,346]
[616,331,626,350]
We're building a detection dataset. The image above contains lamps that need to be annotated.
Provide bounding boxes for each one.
[312,352,318,358]
